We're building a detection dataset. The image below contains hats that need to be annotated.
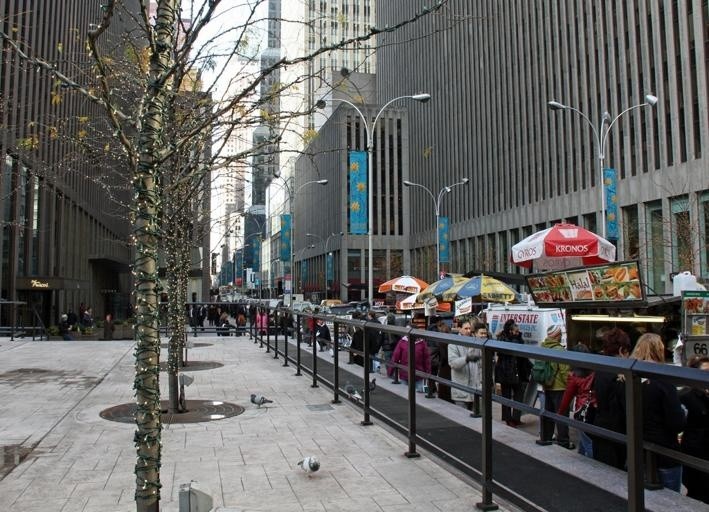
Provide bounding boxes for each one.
[546,325,561,339]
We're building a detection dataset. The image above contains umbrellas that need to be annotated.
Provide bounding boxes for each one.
[511,218,616,271]
[378,272,521,311]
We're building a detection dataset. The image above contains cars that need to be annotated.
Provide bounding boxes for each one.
[292,300,411,340]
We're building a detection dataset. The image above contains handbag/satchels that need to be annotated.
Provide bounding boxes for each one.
[574,401,599,440]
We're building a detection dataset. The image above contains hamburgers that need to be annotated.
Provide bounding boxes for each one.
[594,288,603,299]
[606,286,618,299]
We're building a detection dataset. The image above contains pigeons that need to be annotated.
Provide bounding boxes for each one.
[297,455,321,478]
[361,378,377,395]
[249,393,274,408]
[354,389,362,403]
[344,380,354,397]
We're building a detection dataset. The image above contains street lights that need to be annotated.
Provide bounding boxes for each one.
[220,209,286,300]
[402,178,469,280]
[293,233,344,299]
[273,170,329,308]
[545,94,658,241]
[317,93,432,305]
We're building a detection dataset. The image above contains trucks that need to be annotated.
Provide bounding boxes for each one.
[475,303,567,368]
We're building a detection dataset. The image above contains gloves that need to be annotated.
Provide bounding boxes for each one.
[467,355,479,363]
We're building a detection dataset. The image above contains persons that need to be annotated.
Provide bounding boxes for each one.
[58,301,138,341]
[287,307,385,373]
[186,299,276,337]
[386,299,709,505]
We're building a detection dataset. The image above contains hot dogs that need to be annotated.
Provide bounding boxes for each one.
[615,268,626,282]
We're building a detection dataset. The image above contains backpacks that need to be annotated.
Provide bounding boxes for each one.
[530,344,560,387]
